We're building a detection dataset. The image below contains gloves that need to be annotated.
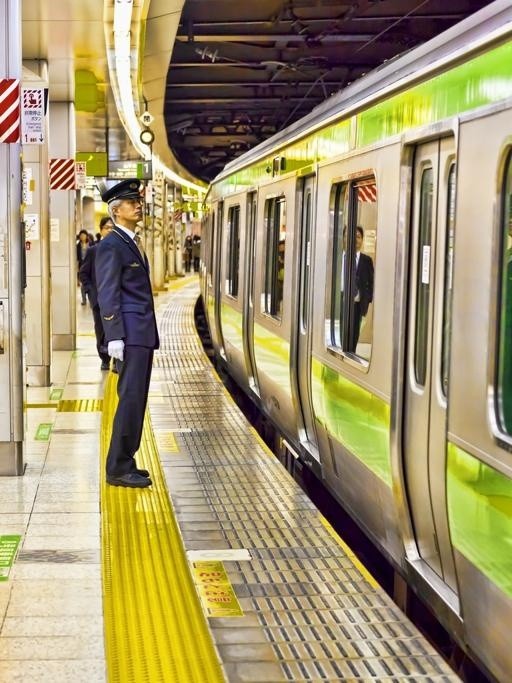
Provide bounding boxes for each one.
[107,339,125,362]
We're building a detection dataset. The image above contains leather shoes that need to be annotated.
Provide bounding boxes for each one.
[107,470,152,488]
[101,362,118,373]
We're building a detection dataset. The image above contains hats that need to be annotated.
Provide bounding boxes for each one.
[102,178,144,205]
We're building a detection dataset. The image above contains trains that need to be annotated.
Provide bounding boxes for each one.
[191,0,511,683]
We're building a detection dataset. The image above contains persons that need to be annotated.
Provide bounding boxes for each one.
[341,224,374,352]
[185,233,200,274]
[95,178,160,488]
[78,216,114,370]
